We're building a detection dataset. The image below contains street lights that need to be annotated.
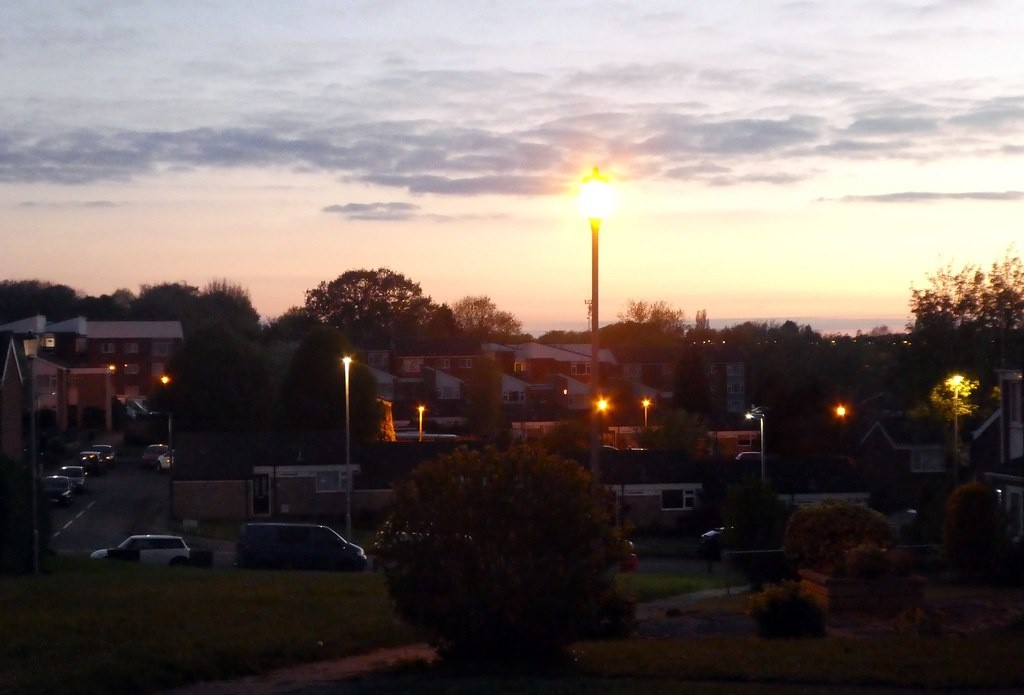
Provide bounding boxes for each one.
[642,398,651,452]
[834,404,850,454]
[951,375,967,488]
[742,411,768,485]
[417,405,426,440]
[341,356,356,551]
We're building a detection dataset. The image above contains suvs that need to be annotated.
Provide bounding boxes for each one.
[91,534,193,571]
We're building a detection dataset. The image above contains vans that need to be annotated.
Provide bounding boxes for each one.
[236,521,369,574]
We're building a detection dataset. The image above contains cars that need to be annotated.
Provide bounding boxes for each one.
[77,450,107,479]
[40,474,74,505]
[698,525,739,550]
[735,451,767,463]
[94,444,117,470]
[58,464,87,494]
[598,444,620,455]
[154,449,177,474]
[141,444,169,469]
